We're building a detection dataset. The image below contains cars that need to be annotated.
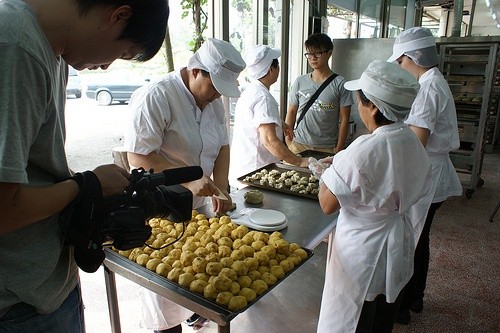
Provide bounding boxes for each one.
[85,72,150,106]
[228,86,243,127]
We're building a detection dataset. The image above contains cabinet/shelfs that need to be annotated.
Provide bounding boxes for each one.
[439,42,500,199]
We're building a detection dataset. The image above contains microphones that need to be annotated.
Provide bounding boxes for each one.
[145,166,203,186]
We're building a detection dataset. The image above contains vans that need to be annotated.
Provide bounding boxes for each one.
[65,66,81,99]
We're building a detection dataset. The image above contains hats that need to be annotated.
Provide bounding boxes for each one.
[344,60,421,109]
[386,27,436,63]
[198,37,246,97]
[244,45,282,74]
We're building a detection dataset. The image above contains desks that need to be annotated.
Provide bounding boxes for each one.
[97,185,341,333]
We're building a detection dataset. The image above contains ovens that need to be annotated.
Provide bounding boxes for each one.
[332,36,500,154]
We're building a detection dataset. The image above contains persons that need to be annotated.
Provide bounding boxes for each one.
[284,33,355,155]
[386,27,464,325]
[229,44,310,190]
[124,38,247,333]
[0,0,169,333]
[308,60,433,333]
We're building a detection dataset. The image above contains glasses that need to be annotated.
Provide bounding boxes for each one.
[304,49,329,58]
